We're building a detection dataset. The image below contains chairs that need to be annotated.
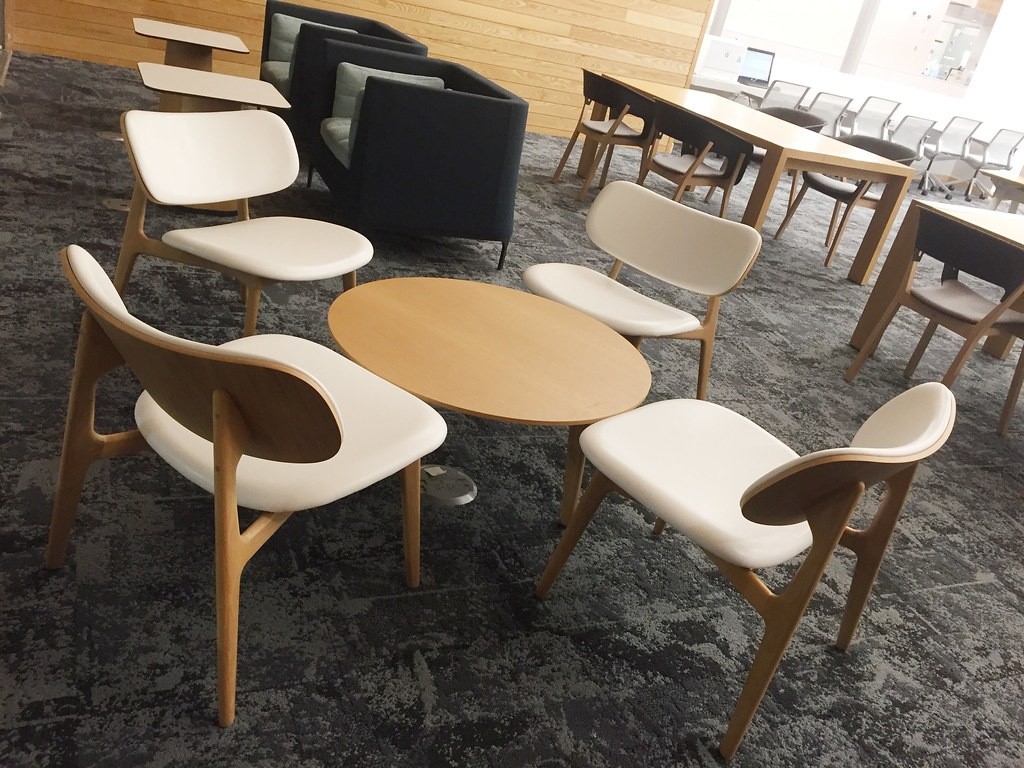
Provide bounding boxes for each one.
[552,67,655,204]
[524,181,763,400]
[790,92,854,176]
[528,382,956,759]
[112,111,375,340]
[760,80,811,110]
[704,107,828,212]
[637,96,754,219]
[909,116,983,199]
[834,96,901,186]
[842,206,1024,435]
[775,135,917,265]
[940,130,1024,202]
[46,244,448,726]
[890,115,936,192]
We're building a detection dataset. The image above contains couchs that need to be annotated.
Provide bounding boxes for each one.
[256,0,428,152]
[306,39,529,269]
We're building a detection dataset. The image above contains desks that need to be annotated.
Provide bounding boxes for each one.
[328,277,653,526]
[848,198,1024,356]
[577,74,916,285]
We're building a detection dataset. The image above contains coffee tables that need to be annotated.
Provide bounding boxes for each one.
[137,62,291,216]
[133,18,250,72]
